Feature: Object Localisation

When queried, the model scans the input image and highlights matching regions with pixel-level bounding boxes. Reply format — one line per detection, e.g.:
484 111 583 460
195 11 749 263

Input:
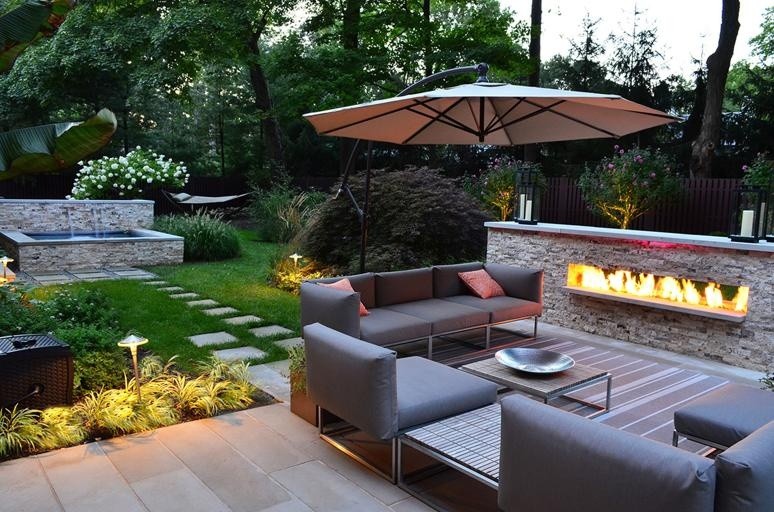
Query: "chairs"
302 320 498 485
498 381 774 512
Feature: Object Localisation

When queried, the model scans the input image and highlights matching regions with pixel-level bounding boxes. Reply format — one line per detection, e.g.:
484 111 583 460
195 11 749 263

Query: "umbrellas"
301 63 683 273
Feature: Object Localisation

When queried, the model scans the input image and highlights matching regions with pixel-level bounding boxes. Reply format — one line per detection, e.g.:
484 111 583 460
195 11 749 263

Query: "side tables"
397 402 501 512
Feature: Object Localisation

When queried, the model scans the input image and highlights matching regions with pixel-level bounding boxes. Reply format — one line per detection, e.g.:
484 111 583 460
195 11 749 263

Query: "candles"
519 193 532 221
758 202 766 237
740 209 754 236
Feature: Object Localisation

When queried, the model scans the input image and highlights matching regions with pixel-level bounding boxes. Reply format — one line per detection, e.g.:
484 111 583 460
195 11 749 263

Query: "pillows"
172 192 192 201
315 278 370 316
457 268 506 299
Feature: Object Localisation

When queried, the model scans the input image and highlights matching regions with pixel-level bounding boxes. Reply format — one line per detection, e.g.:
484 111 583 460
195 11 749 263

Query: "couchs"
301 261 545 361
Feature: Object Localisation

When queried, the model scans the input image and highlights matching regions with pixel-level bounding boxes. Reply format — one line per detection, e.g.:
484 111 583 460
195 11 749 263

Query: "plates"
495 347 575 375
